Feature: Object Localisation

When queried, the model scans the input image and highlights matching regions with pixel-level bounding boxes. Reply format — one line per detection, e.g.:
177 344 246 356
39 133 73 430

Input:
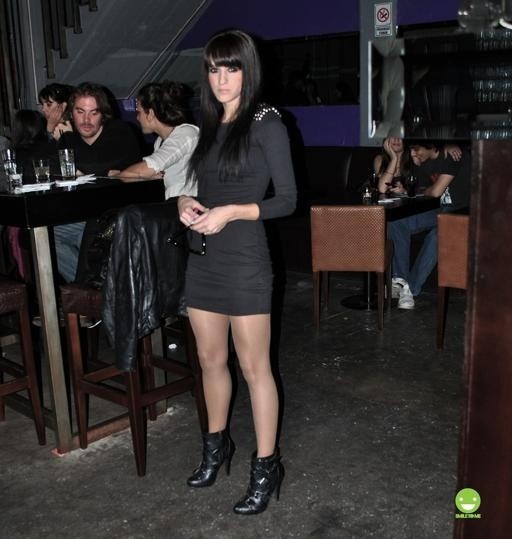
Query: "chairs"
310 205 394 330
437 213 469 351
59 200 208 477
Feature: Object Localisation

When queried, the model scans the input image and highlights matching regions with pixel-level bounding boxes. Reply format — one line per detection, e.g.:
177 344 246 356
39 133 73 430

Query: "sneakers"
385 277 407 299
79 315 102 328
32 306 65 327
397 284 415 310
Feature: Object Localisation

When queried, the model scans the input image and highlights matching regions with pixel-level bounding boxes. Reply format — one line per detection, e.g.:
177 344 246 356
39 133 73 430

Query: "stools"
0 282 46 445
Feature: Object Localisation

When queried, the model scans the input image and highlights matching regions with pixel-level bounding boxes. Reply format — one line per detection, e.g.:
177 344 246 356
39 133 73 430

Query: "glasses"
184 223 206 256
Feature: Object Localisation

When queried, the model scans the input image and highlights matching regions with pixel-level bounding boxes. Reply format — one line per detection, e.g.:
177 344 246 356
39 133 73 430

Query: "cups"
63 178 77 191
57 149 76 177
32 159 50 179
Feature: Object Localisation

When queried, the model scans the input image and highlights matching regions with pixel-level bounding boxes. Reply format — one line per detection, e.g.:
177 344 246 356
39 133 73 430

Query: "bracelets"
387 170 393 174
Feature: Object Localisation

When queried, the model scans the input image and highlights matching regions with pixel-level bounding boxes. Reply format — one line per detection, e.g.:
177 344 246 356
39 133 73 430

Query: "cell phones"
385 182 398 188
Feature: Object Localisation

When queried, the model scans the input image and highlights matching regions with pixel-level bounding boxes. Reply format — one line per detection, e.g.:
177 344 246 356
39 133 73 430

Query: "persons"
384 140 473 310
178 30 296 516
373 137 462 194
1 110 57 285
108 83 203 200
39 82 73 141
31 81 146 328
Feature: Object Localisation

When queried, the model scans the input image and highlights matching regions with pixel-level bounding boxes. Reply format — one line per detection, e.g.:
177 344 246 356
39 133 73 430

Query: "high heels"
233 445 285 515
186 429 235 488
